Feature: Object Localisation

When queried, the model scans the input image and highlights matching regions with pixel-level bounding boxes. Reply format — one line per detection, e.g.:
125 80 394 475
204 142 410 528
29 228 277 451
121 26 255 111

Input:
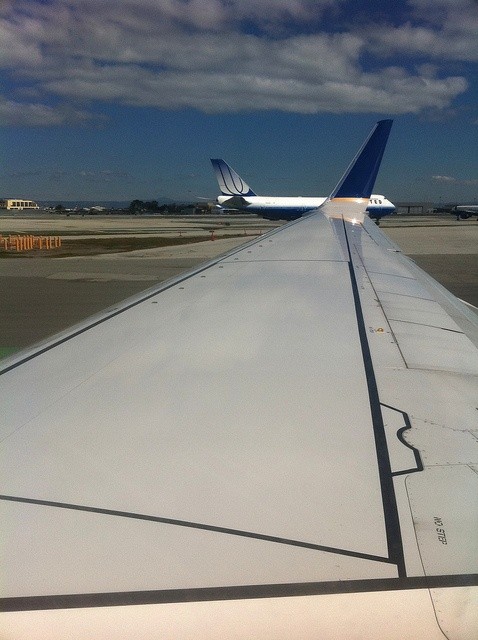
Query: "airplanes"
209 157 398 227
431 200 478 224
0 113 476 640
